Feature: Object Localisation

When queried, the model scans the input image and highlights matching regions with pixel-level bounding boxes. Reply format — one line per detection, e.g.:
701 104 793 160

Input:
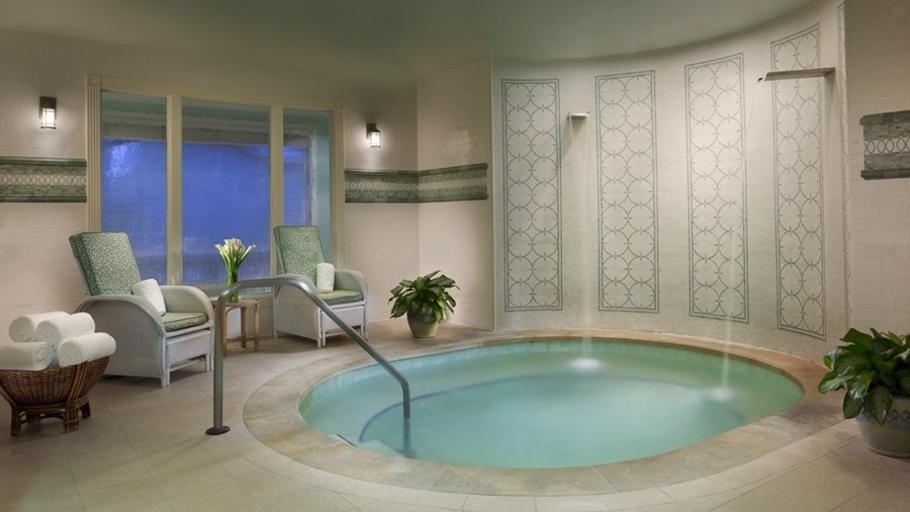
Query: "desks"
214 297 262 357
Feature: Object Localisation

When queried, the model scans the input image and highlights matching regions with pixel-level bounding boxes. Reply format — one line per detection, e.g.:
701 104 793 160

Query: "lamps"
363 120 386 153
36 94 61 132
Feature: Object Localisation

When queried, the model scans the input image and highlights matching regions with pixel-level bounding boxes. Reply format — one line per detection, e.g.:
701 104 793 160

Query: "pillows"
132 278 167 316
316 261 334 293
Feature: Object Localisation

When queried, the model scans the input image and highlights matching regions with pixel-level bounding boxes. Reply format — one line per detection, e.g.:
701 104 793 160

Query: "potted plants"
819 327 910 459
386 269 461 338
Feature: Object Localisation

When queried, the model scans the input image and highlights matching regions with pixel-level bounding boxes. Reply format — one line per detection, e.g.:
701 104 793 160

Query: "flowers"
213 237 256 266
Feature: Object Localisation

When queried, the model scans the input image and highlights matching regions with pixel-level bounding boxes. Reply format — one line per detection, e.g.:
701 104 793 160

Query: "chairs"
271 228 368 348
69 232 214 387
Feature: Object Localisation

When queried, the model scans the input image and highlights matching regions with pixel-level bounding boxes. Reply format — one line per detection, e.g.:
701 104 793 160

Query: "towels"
0 310 117 372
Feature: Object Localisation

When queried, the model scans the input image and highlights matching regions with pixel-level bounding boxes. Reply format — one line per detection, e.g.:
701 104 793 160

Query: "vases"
226 268 241 302
